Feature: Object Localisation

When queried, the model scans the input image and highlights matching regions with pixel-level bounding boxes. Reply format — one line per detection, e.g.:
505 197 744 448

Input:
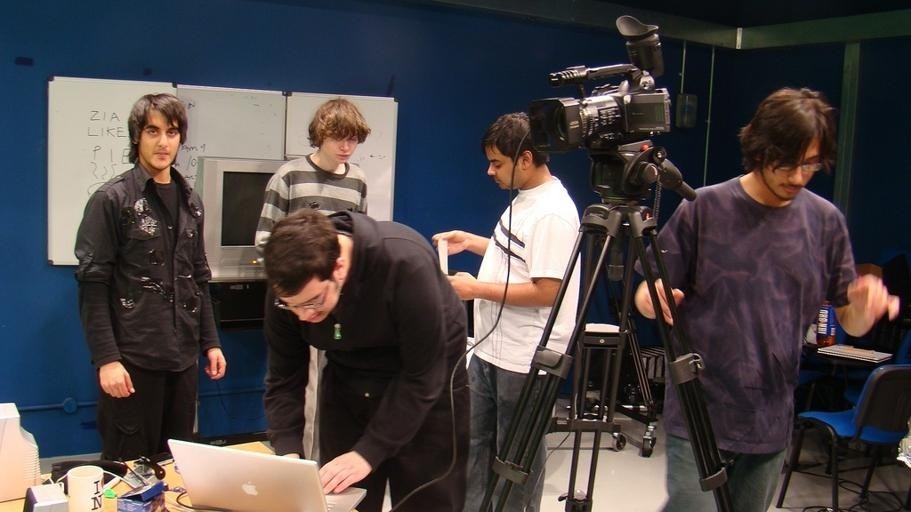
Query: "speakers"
675 94 698 129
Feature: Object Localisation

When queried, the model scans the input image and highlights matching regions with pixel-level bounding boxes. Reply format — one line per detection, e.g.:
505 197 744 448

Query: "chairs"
776 316 911 511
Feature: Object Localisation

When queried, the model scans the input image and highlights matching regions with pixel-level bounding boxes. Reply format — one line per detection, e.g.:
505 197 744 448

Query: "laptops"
167 436 367 512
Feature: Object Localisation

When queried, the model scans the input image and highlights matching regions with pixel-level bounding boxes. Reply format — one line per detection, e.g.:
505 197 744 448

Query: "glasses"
767 154 824 174
274 281 332 312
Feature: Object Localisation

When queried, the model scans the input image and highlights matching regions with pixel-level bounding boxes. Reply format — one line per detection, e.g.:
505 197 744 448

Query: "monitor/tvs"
195 157 291 280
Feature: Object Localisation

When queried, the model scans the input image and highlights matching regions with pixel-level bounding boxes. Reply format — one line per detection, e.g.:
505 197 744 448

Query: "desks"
1 439 278 512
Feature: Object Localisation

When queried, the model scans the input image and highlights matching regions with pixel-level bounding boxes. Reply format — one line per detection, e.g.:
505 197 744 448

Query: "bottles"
818 299 837 348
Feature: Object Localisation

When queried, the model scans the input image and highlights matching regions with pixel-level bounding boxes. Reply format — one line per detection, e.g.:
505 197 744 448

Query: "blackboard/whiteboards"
174 84 287 199
47 75 177 265
285 92 400 222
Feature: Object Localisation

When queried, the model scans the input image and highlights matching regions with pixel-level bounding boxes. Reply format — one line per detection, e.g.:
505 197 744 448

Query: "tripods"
478 203 736 512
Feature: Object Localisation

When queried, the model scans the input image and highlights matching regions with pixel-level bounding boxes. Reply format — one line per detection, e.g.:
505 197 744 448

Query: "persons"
255 98 370 458
264 208 474 511
635 87 901 511
76 93 230 459
434 109 586 510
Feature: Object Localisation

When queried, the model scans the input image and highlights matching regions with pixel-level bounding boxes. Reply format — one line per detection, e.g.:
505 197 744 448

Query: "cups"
67 466 105 511
